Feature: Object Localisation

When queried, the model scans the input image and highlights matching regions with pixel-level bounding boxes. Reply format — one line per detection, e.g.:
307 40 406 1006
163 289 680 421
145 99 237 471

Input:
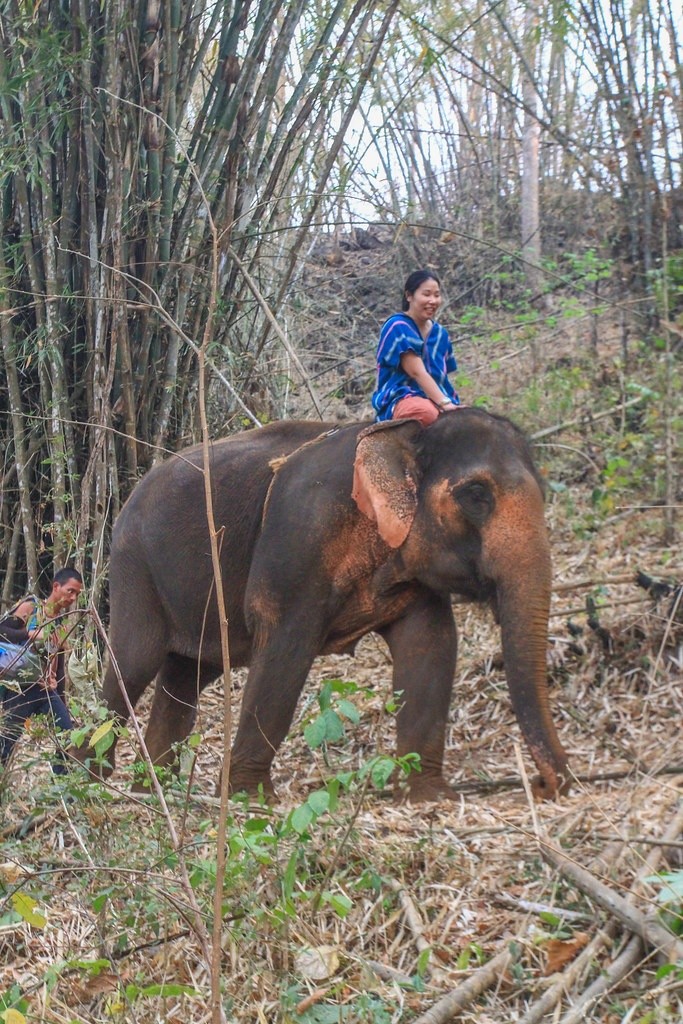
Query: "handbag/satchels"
0 594 45 686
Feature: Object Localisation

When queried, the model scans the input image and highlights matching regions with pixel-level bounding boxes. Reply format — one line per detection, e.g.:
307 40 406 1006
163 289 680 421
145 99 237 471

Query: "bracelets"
438 397 451 406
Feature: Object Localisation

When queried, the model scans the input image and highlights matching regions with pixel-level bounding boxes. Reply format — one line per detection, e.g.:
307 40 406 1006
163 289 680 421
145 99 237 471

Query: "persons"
0 567 82 773
370 270 471 421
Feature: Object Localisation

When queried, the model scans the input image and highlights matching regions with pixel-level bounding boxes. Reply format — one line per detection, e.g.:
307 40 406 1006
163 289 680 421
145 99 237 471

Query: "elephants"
66 408 574 815
